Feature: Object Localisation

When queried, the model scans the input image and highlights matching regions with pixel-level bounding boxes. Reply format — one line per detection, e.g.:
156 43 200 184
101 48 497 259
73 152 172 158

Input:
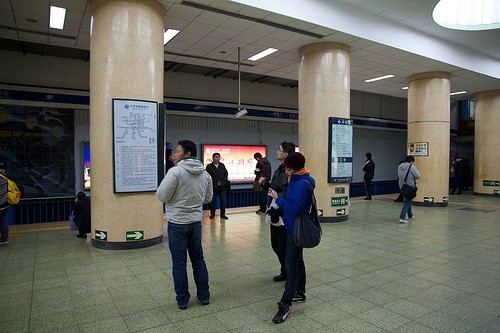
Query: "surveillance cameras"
235 109 248 118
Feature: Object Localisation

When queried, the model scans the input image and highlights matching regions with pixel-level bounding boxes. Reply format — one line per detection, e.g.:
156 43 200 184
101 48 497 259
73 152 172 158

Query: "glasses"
276 148 283 152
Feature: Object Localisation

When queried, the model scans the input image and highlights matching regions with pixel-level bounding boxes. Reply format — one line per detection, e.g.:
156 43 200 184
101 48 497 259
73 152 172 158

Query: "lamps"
235 47 248 118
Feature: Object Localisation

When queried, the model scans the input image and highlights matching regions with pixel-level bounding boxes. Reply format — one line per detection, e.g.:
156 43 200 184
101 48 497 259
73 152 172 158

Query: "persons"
206 153 228 219
166 149 175 174
73 192 91 238
398 155 421 223
268 152 315 323
157 140 214 310
363 152 375 200
268 142 295 282
254 153 271 214
0 176 10 246
449 156 471 195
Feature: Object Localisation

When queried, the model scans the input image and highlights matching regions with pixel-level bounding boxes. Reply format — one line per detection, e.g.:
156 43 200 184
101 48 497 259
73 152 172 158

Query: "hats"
282 152 305 170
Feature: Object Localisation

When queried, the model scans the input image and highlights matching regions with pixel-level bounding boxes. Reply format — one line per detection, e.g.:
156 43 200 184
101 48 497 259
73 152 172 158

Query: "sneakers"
273 305 290 323
290 292 306 302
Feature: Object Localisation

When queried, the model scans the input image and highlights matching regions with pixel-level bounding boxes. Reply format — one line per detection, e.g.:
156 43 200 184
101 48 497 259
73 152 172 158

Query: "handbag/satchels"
253 180 262 192
401 184 418 198
294 178 321 249
225 181 231 195
70 210 78 230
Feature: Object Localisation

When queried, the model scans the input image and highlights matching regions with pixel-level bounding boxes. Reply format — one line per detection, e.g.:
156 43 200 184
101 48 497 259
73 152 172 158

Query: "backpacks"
0 174 21 204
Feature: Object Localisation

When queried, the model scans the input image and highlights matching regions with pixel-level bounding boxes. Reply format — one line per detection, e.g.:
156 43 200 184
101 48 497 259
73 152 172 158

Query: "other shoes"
364 196 372 200
273 275 287 282
0 240 8 245
179 305 187 309
210 213 215 219
409 216 414 221
77 233 86 238
221 214 227 219
256 209 262 214
399 219 407 223
201 300 209 305
